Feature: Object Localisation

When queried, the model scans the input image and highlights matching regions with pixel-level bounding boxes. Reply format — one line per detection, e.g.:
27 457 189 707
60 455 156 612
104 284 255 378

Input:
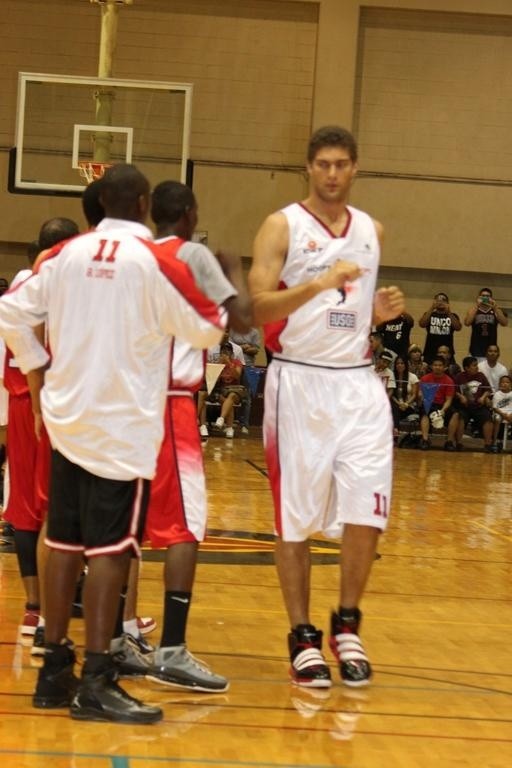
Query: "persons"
193 322 273 438
248 126 405 687
0 163 252 724
368 288 511 454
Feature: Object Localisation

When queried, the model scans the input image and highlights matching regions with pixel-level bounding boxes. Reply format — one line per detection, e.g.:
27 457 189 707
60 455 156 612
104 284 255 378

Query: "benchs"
200 398 243 438
400 415 512 453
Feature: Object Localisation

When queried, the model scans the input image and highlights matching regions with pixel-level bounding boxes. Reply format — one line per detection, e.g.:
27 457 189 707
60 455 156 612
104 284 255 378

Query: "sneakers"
288 624 331 688
70 666 161 723
327 608 373 686
33 666 78 709
421 439 430 449
109 633 156 679
200 417 249 438
21 572 157 656
446 441 500 453
147 643 230 692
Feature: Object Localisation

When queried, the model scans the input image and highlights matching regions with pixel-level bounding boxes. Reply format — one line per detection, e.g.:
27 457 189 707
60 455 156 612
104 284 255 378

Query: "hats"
408 344 422 355
380 351 393 362
221 342 233 350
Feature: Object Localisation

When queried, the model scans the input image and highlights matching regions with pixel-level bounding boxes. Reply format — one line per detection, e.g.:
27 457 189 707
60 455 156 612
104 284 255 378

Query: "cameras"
221 350 230 359
482 296 490 304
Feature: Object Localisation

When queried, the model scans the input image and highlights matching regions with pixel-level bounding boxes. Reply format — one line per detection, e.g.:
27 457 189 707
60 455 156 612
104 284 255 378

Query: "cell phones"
438 295 443 302
